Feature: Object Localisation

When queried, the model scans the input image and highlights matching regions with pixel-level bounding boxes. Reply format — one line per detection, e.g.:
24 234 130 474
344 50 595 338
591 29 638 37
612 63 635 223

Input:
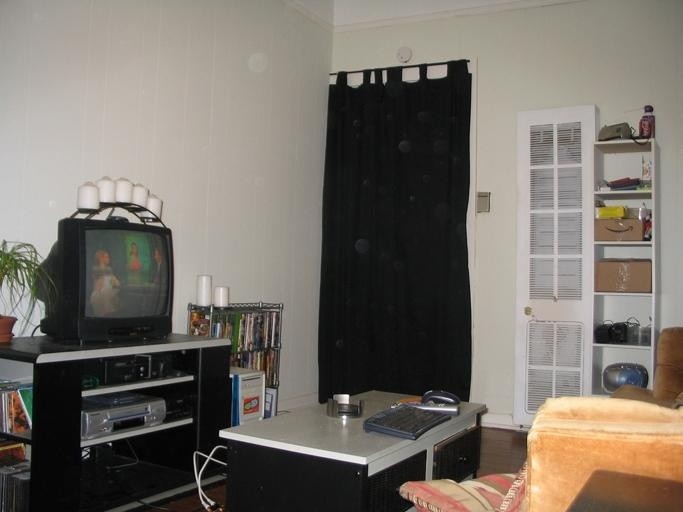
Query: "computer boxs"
229 366 265 427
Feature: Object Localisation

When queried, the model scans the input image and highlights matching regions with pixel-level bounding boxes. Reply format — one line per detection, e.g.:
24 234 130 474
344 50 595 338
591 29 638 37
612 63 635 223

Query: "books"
0 379 34 511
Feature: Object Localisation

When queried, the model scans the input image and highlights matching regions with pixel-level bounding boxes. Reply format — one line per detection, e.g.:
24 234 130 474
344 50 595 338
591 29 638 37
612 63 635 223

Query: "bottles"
638 104 655 138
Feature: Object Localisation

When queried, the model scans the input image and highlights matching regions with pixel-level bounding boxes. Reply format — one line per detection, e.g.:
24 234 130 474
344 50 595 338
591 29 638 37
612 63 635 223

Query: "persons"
125 240 145 280
90 249 122 315
149 244 168 284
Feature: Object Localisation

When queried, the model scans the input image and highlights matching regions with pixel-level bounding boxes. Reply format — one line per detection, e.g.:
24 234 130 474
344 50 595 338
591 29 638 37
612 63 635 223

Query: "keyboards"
364 406 451 440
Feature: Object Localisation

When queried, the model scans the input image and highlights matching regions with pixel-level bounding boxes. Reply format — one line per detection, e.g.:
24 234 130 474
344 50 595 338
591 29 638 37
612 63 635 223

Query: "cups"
77 176 163 219
196 275 229 308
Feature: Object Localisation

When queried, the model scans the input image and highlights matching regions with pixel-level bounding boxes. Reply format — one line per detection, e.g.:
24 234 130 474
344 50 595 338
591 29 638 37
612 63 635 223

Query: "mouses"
422 390 459 404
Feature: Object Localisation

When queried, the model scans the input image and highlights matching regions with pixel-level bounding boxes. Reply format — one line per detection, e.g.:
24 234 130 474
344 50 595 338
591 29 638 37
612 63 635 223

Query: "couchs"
520 327 683 512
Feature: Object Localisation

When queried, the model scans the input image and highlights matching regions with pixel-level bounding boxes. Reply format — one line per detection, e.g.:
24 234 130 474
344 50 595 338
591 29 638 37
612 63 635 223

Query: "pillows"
498 459 526 512
399 472 518 512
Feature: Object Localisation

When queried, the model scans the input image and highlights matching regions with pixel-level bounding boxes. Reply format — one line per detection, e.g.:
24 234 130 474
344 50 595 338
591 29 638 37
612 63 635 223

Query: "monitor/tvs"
34 218 173 344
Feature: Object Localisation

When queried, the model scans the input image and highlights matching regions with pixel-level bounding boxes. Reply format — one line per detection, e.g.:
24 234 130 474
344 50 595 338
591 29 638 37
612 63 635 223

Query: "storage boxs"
595 258 652 293
594 218 645 241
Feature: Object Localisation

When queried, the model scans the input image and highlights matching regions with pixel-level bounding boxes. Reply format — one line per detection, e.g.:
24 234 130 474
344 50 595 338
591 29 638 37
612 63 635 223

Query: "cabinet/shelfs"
594 139 662 397
516 106 600 427
0 334 231 512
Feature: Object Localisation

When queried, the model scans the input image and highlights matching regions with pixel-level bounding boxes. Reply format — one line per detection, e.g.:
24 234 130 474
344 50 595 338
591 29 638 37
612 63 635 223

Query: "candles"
115 177 133 202
77 181 100 209
214 287 229 307
132 182 149 208
140 194 163 222
96 177 117 203
195 275 211 306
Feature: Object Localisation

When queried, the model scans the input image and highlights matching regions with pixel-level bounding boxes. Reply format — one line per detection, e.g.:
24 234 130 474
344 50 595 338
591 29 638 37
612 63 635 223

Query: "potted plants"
0 238 58 343
